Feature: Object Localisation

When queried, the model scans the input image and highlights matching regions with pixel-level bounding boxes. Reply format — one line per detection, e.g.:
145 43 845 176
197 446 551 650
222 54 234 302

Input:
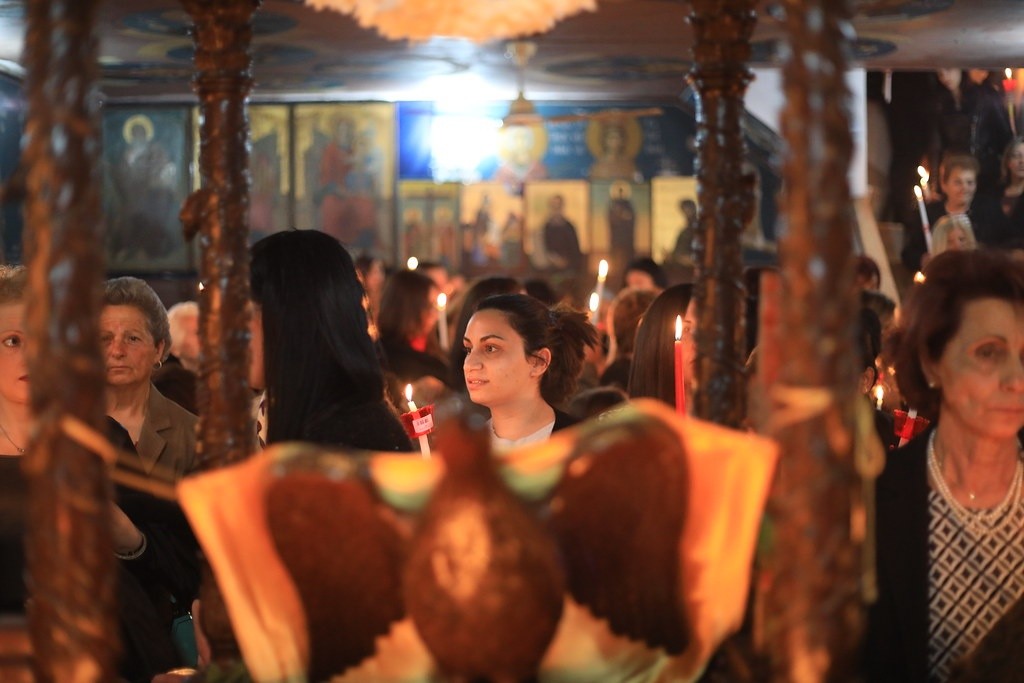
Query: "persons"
0 66 1024 683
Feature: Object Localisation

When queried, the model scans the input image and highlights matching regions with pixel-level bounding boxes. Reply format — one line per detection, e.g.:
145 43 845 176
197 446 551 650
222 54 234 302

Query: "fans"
401 42 664 126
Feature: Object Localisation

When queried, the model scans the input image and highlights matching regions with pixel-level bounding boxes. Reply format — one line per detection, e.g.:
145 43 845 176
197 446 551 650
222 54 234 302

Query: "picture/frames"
0 71 400 270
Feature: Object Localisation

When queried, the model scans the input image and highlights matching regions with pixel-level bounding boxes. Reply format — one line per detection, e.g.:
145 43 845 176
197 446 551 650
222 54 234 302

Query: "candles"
914 185 931 252
918 166 930 195
437 292 449 350
875 385 883 410
898 409 917 447
674 315 685 414
589 259 608 324
406 383 430 456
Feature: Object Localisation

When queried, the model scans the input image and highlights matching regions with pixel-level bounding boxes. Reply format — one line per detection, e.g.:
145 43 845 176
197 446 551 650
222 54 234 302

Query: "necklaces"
0 424 25 452
926 451 1023 533
929 428 1021 519
